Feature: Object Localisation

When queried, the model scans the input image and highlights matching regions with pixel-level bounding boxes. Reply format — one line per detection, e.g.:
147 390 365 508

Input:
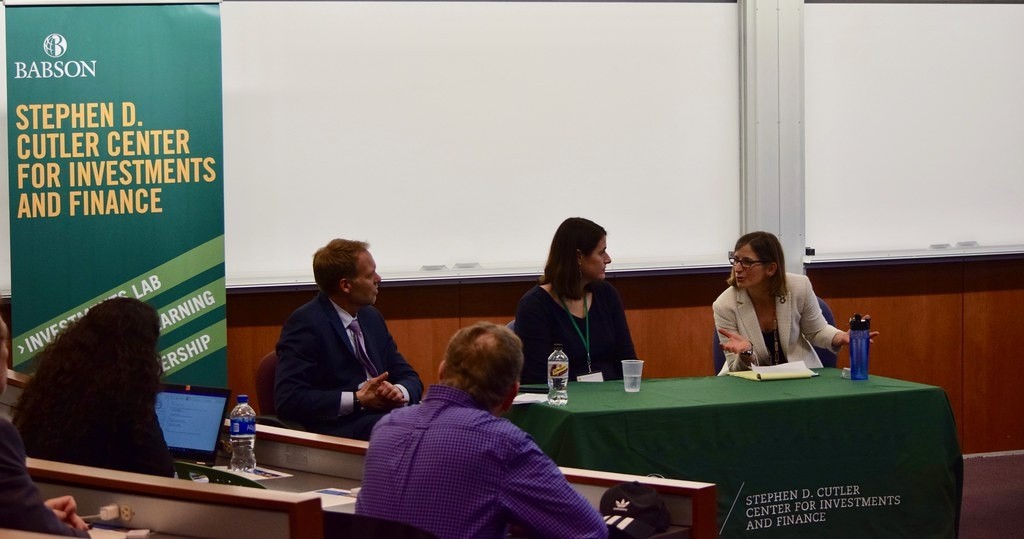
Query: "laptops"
154 383 232 466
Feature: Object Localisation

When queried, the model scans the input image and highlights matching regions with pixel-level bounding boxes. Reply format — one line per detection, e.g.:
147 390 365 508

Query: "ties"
348 320 377 377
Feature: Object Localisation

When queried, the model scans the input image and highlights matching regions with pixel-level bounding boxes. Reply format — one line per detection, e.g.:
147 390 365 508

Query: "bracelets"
354 394 364 413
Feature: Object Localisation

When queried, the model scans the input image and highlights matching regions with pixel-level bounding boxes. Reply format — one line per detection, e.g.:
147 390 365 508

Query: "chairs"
166 296 833 539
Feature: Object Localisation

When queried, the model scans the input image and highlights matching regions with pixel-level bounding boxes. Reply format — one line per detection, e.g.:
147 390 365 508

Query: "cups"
622 359 644 392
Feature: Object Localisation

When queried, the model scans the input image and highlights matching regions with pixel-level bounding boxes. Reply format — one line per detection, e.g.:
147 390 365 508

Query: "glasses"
729 257 761 267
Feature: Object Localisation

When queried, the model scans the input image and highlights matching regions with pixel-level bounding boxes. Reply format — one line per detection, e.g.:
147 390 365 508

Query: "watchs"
742 342 753 356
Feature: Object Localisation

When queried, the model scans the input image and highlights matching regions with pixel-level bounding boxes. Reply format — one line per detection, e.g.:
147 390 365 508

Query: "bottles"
230 395 256 472
547 344 569 406
850 313 870 380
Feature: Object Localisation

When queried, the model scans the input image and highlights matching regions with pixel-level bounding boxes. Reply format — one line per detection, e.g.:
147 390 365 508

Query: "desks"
0 370 719 539
504 366 963 539
23 457 323 538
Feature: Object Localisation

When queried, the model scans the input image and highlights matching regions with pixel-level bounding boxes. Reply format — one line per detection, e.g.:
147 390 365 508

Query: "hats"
599 481 670 539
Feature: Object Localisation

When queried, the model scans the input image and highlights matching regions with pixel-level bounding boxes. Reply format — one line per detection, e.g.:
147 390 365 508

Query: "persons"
270 239 425 440
514 218 637 381
712 231 880 376
0 317 93 539
13 298 176 475
356 322 608 539
